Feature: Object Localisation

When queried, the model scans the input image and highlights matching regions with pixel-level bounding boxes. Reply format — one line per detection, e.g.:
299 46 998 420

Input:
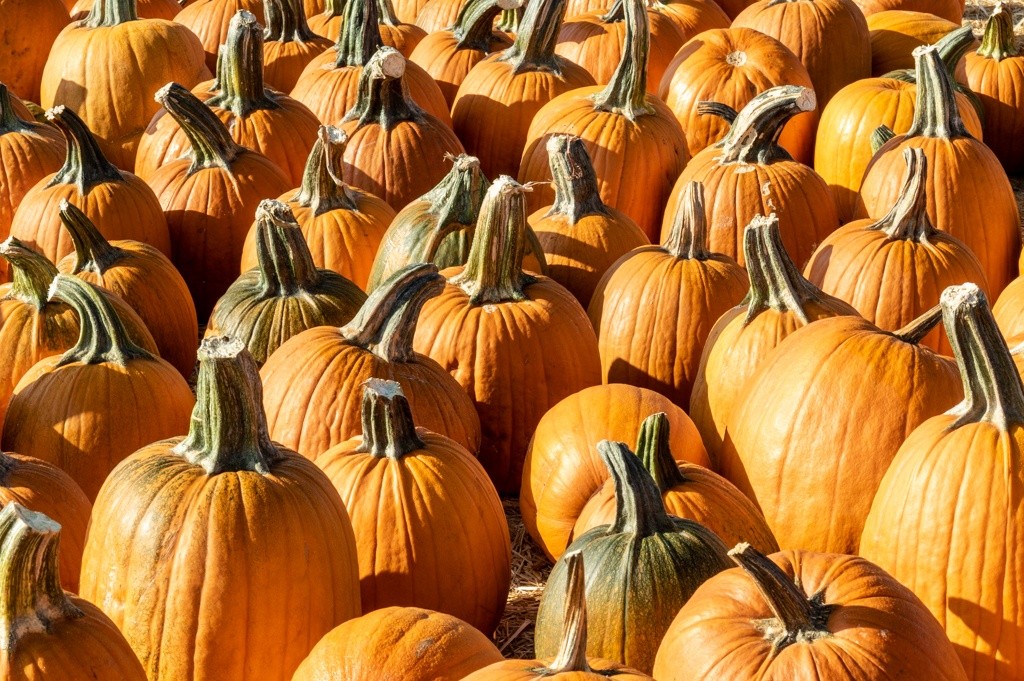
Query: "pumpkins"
0 1 1024 680
